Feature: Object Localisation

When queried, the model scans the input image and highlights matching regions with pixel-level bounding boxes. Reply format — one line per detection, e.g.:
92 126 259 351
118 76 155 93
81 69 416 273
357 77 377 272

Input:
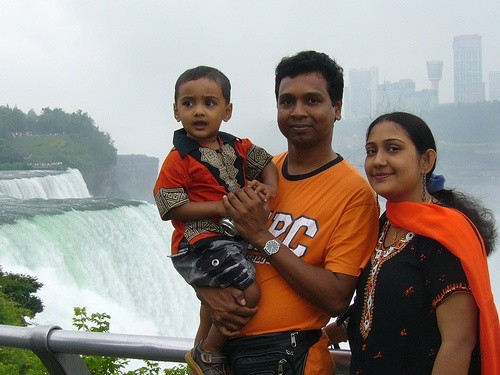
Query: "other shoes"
184 340 225 375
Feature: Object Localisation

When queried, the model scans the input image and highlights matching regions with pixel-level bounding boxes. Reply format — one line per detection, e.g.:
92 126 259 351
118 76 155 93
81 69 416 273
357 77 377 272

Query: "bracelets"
321 327 332 345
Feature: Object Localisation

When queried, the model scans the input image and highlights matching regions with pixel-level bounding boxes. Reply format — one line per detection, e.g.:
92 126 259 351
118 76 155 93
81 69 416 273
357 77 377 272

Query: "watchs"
260 236 282 258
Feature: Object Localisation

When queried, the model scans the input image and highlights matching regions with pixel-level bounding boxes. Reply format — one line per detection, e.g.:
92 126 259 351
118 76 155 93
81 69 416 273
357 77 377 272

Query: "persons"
192 50 381 375
155 66 279 375
321 112 500 374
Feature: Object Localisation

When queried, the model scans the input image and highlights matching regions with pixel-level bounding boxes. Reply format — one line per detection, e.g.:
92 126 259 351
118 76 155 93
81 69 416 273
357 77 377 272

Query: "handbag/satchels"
224 330 320 375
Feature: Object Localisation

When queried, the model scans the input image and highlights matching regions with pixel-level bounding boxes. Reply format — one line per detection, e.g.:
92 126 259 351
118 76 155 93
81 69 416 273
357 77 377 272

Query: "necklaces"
382 193 432 241
